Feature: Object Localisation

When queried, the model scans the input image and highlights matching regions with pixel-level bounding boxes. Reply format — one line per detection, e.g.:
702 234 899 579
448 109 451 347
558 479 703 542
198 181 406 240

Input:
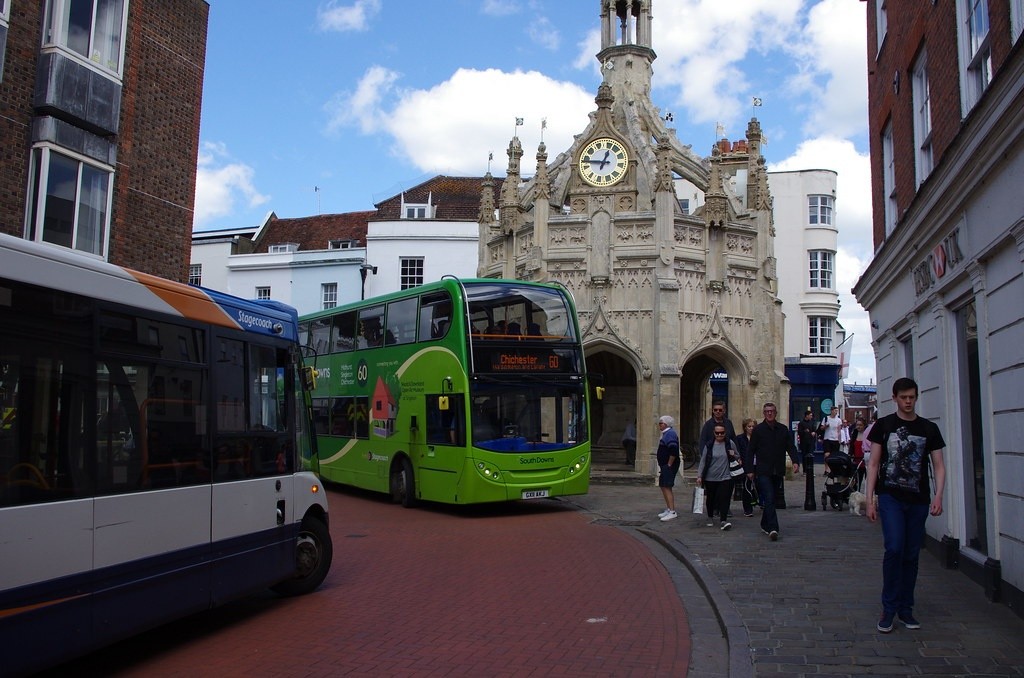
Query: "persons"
657 416 680 520
622 419 636 464
868 378 947 632
798 407 877 493
697 401 801 540
450 393 474 444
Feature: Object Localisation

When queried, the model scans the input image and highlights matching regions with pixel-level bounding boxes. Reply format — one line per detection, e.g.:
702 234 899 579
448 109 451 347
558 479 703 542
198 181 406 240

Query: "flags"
717 125 724 133
516 118 523 126
837 337 853 378
754 98 762 106
762 136 767 145
666 112 673 123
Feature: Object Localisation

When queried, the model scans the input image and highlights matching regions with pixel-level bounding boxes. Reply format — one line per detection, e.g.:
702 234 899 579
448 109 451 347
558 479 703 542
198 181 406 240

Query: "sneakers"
877 611 896 632
719 521 732 530
658 509 671 518
705 517 714 526
898 613 921 628
660 511 678 521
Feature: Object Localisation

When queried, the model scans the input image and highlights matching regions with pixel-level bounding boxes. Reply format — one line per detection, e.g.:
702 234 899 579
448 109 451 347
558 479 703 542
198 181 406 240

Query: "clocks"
579 137 629 188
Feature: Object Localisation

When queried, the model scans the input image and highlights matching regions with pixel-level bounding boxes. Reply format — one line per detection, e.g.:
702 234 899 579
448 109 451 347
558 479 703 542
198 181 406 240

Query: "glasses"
715 431 725 435
714 409 722 412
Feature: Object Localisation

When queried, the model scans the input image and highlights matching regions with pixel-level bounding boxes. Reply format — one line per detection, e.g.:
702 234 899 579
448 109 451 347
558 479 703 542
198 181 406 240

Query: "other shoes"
744 511 754 517
823 470 829 477
769 531 778 541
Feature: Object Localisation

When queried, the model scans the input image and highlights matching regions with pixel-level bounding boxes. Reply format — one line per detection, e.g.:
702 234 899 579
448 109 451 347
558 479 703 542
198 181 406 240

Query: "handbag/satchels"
692 481 704 514
728 454 744 476
816 416 828 439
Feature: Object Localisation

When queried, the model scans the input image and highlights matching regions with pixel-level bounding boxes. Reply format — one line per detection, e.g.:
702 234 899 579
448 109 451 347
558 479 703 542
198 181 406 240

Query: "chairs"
420 317 544 342
0 441 166 506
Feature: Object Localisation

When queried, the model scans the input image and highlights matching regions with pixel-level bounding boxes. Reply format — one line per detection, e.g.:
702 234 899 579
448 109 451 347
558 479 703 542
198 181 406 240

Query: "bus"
269 273 608 508
0 231 335 676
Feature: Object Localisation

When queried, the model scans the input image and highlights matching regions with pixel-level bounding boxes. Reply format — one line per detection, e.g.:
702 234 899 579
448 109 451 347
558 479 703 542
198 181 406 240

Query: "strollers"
817 451 866 510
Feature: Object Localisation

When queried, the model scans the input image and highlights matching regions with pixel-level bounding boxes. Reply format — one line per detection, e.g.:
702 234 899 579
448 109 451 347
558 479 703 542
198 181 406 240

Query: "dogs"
849 491 879 516
